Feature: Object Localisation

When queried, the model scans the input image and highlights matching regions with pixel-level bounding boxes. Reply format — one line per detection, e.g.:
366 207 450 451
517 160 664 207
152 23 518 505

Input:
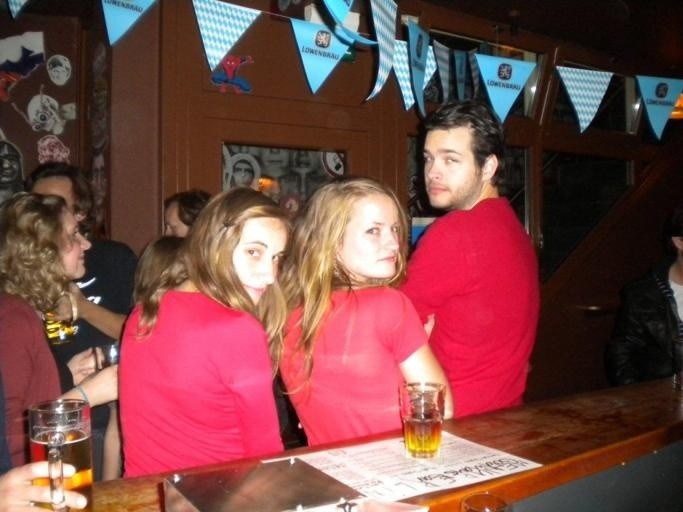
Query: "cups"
397 381 447 457
100 340 121 368
44 305 79 348
27 398 95 511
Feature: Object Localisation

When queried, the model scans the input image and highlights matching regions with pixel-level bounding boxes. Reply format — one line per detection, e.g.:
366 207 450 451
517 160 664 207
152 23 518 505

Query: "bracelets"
76 384 88 405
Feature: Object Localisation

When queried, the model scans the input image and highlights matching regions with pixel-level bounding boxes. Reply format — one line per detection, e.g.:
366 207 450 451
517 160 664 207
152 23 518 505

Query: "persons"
23 162 138 483
101 236 190 486
233 160 253 190
0 191 118 470
290 150 322 175
392 99 541 418
160 191 211 241
603 204 683 389
277 178 454 446
324 152 344 176
0 459 93 512
259 147 290 177
119 183 294 482
0 140 22 186
